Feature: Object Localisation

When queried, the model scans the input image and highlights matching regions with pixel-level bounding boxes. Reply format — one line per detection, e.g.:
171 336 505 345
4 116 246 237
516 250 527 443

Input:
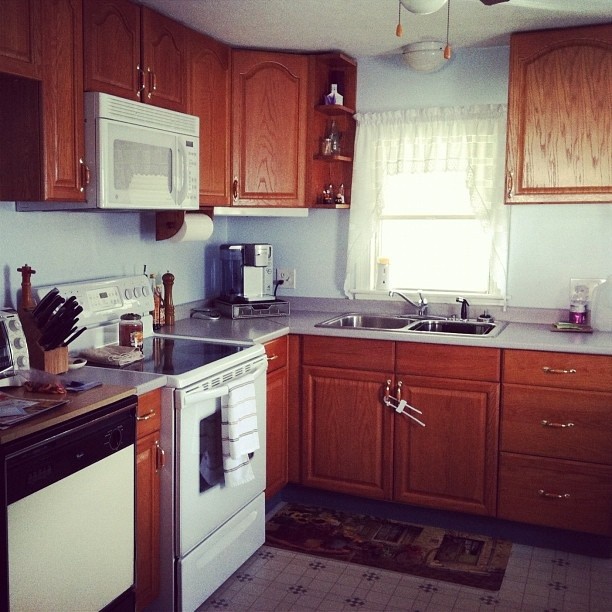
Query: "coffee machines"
220 241 275 302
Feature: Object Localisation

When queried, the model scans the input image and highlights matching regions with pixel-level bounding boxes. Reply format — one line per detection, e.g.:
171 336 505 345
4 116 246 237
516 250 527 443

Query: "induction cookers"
15 275 265 388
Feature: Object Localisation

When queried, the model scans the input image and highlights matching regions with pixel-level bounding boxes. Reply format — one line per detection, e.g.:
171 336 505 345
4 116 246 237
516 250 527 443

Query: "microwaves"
15 91 201 212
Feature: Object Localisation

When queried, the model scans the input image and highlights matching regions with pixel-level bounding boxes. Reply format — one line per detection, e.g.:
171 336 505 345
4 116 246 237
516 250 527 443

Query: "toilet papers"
166 214 214 242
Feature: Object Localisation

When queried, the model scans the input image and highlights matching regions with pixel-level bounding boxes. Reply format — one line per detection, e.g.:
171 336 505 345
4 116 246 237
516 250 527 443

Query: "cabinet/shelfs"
289 332 499 519
501 351 610 551
85 0 188 110
304 46 357 215
265 336 289 509
503 26 612 204
1 1 86 208
231 43 316 203
187 35 231 203
133 388 167 612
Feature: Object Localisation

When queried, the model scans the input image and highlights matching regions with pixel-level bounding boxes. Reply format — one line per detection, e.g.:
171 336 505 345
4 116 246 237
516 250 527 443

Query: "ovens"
159 355 266 611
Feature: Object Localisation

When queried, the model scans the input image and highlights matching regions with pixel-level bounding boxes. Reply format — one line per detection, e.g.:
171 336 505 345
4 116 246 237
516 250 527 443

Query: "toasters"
0 310 31 389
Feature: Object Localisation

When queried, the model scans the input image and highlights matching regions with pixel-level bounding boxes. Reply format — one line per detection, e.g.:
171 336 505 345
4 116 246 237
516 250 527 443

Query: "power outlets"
276 268 296 289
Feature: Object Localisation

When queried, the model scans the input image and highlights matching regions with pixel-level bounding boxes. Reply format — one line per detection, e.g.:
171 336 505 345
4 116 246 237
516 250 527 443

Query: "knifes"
32 287 87 350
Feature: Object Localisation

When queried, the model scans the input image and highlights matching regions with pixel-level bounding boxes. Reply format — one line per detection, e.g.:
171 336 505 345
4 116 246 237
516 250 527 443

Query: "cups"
376 258 390 291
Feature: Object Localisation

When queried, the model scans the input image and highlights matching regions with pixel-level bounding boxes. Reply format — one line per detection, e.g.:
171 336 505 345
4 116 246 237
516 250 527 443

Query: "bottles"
118 313 144 350
148 273 160 332
157 285 166 326
329 118 341 155
325 83 344 107
569 303 586 324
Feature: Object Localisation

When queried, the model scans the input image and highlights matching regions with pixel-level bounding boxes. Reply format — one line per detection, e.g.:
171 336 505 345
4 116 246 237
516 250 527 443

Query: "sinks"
314 313 417 337
408 318 511 337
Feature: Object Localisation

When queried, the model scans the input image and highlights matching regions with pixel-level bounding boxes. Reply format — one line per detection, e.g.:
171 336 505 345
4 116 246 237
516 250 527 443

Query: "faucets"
389 290 431 320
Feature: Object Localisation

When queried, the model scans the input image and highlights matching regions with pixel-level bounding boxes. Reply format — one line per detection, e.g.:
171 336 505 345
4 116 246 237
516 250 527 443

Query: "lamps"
398 0 448 17
403 40 453 74
